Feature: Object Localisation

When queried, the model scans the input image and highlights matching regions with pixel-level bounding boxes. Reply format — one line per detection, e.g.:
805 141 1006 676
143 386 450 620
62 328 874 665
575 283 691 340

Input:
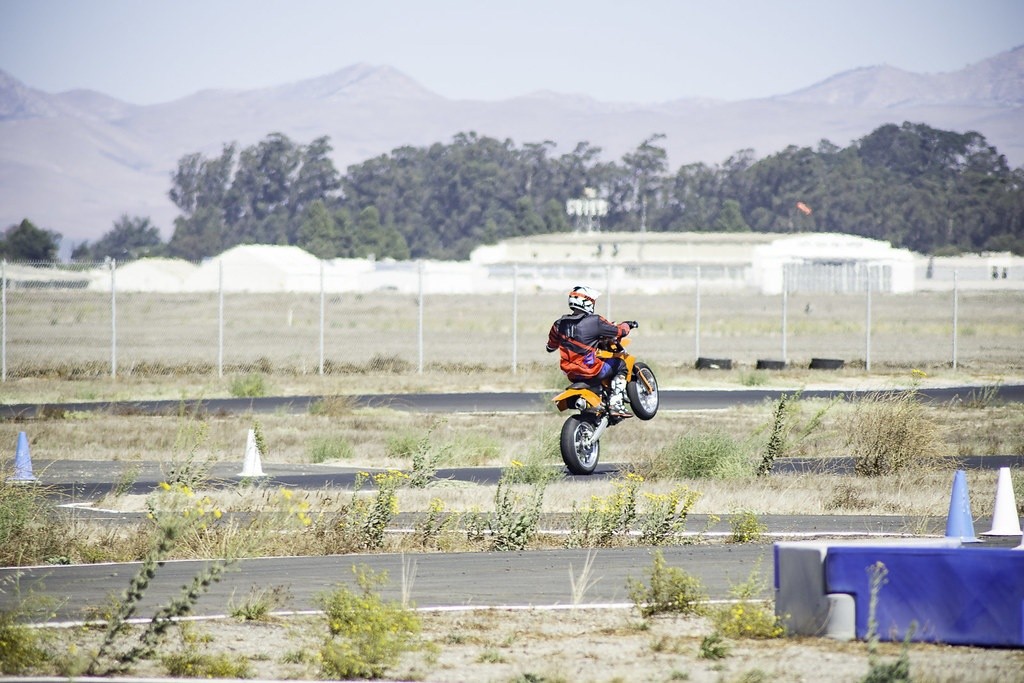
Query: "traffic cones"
2 430 38 481
945 470 987 544
979 466 1024 537
1011 529 1024 551
236 429 268 477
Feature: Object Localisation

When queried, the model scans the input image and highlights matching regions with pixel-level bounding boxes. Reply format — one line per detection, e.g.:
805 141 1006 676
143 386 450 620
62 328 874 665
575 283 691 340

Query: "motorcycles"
549 320 661 475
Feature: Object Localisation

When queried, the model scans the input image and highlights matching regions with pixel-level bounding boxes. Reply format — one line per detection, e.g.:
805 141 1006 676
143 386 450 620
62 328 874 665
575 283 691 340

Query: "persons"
545 285 634 415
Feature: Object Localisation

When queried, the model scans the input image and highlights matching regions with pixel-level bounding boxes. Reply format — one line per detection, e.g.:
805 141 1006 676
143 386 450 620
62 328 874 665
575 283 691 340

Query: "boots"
609 375 634 417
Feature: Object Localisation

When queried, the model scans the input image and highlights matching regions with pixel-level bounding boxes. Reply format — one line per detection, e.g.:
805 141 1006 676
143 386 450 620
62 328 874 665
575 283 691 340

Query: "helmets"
569 285 603 314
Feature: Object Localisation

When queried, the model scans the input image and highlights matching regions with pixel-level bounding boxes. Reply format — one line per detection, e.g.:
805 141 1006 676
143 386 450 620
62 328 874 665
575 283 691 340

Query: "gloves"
625 321 635 328
